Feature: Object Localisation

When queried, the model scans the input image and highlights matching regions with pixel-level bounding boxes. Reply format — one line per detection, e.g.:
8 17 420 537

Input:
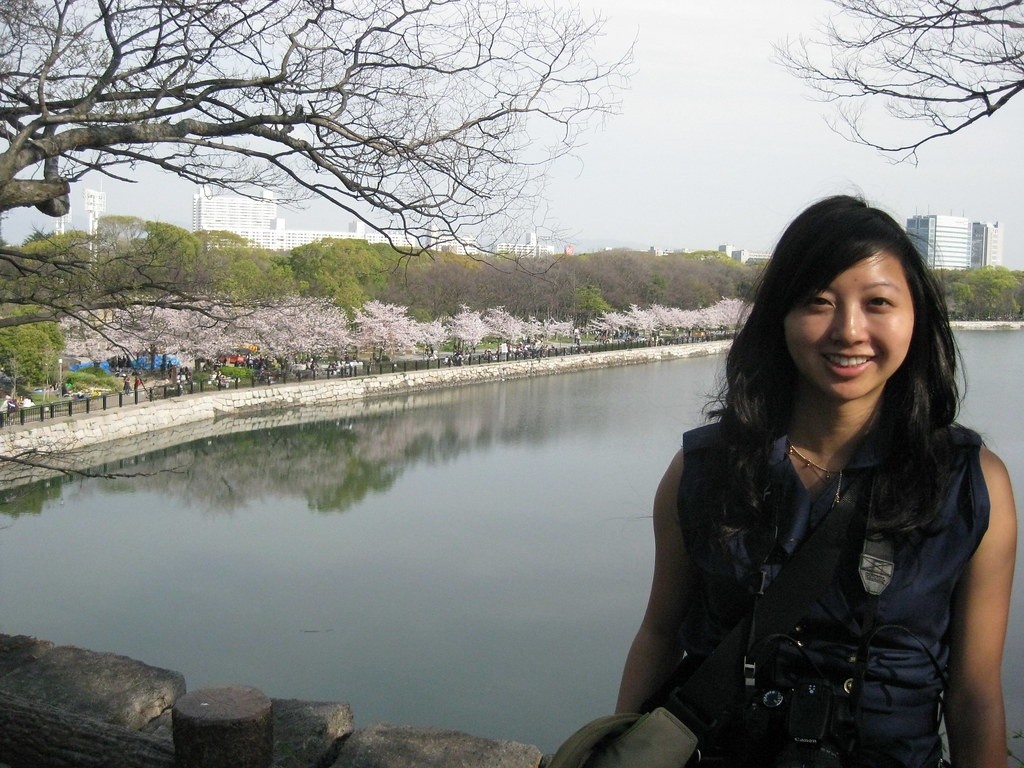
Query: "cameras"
727 640 861 768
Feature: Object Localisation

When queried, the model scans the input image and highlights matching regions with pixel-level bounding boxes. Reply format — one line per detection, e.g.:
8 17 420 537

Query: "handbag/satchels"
537 710 700 768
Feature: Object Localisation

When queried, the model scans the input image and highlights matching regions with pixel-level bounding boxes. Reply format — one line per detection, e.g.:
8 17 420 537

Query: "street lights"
57 358 63 399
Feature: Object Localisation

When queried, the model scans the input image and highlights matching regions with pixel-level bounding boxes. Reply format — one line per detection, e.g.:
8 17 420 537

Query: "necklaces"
786 438 839 480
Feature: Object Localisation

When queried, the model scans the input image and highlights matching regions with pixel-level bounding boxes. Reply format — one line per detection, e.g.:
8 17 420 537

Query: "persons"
5 393 26 428
168 327 726 396
614 192 1017 766
134 376 141 391
123 376 132 394
